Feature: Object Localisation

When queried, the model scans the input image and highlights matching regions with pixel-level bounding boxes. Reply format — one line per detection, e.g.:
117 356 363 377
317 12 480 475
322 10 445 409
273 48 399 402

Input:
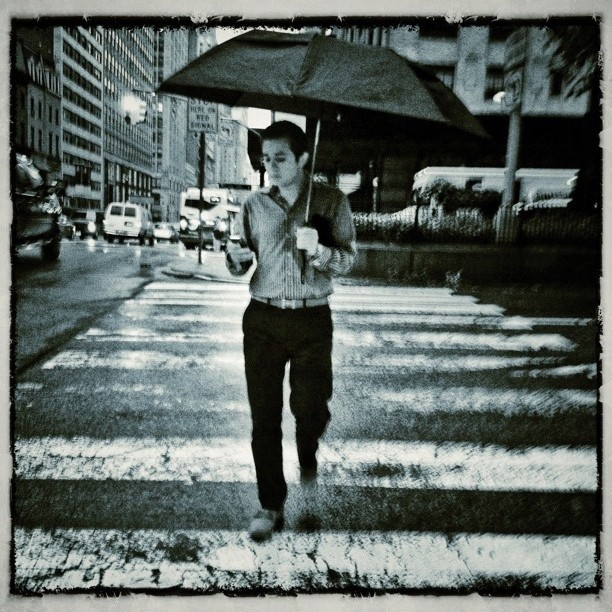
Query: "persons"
222 121 360 542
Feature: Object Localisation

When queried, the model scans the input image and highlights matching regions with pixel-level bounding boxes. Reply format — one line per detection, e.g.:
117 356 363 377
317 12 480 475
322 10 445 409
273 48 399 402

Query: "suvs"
10 153 68 268
66 210 104 241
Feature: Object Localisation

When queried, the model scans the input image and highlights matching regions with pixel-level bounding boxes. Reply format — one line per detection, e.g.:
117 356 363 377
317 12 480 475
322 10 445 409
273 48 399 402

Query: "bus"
178 187 252 251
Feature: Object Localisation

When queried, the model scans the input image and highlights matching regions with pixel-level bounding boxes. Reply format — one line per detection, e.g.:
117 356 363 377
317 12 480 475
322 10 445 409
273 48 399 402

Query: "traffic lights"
119 93 146 126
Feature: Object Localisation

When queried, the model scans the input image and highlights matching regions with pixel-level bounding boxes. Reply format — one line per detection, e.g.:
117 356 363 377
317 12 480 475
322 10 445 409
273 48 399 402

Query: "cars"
152 224 178 245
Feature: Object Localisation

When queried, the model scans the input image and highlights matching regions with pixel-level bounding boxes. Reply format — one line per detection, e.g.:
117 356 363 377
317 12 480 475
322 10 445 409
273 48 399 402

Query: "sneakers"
248 508 285 538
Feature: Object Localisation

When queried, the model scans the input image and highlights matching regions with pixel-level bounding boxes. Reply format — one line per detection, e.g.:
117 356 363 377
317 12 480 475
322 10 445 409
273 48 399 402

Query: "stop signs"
186 96 219 134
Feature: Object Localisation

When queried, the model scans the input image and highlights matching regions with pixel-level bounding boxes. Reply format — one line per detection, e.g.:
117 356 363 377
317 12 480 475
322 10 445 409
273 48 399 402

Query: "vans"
102 200 155 247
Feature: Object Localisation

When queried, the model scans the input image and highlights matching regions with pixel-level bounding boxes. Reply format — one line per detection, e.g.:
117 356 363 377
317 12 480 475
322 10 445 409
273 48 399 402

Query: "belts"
250 294 328 310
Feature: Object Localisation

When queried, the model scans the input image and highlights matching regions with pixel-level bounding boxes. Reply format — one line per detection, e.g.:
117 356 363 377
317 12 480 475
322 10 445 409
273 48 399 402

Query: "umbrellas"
153 30 493 284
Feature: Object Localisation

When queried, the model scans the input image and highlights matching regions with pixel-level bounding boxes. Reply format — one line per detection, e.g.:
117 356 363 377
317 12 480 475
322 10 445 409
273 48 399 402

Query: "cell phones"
228 236 253 268
